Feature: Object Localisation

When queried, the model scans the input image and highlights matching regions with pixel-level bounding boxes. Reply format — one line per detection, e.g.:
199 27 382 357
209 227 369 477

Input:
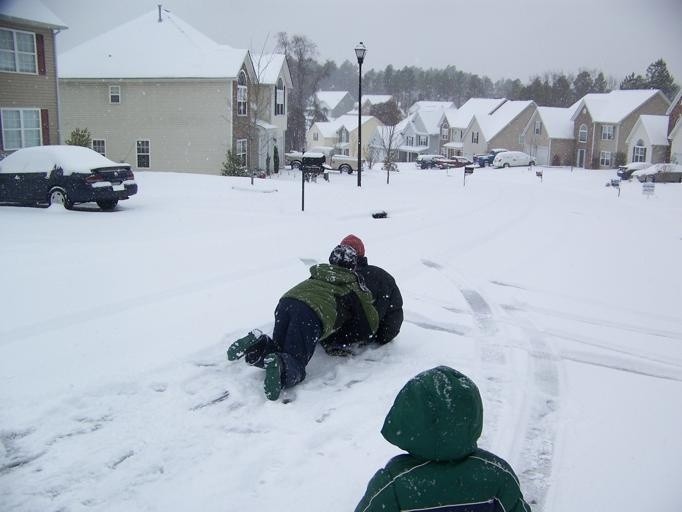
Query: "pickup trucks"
436 156 470 169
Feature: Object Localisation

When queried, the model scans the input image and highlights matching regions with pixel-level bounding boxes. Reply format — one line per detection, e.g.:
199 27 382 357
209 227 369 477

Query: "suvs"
479 149 507 167
300 153 326 175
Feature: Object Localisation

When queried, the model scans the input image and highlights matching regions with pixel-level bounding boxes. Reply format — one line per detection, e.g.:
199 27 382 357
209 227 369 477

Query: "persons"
353 365 531 511
324 234 403 354
224 243 382 404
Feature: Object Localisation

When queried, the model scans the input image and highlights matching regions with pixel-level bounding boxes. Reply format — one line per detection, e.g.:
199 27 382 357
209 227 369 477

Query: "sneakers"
264 353 284 401
227 329 262 360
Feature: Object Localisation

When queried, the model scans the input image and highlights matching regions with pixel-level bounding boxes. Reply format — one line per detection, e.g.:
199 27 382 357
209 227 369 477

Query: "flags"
611 178 622 189
465 165 475 175
535 168 544 177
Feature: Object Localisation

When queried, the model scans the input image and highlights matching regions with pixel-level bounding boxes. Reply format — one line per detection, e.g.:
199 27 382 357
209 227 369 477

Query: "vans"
416 154 446 169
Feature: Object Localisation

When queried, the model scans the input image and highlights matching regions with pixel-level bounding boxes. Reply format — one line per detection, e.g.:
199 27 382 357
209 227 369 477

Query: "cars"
617 161 682 183
493 151 535 167
1 145 137 211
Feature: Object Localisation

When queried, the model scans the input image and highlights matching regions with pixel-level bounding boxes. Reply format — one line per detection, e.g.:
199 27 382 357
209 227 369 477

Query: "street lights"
354 42 366 188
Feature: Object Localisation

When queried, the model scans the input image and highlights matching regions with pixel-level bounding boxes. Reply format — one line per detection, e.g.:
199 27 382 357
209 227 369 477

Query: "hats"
329 245 356 270
341 234 364 257
